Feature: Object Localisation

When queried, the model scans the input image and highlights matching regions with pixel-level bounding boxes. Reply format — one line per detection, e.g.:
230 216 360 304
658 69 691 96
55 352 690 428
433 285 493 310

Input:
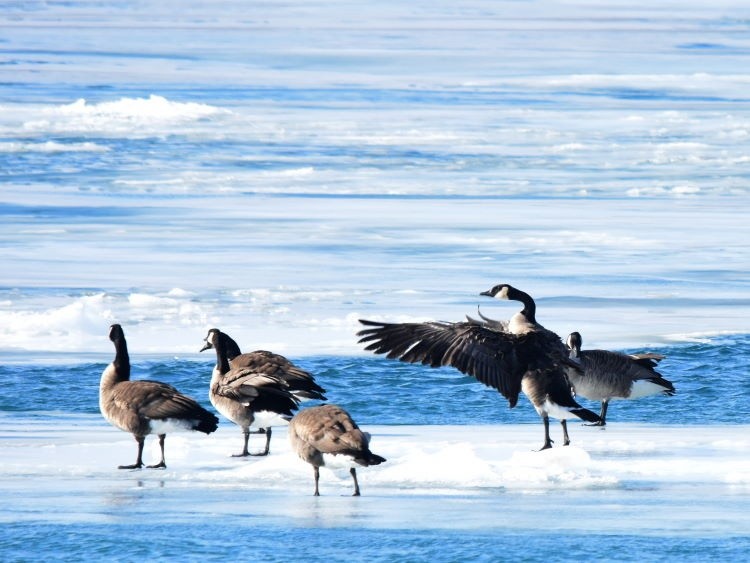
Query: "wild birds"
290 404 387 495
98 324 220 469
356 284 603 451
200 332 329 433
566 332 676 427
204 329 301 458
